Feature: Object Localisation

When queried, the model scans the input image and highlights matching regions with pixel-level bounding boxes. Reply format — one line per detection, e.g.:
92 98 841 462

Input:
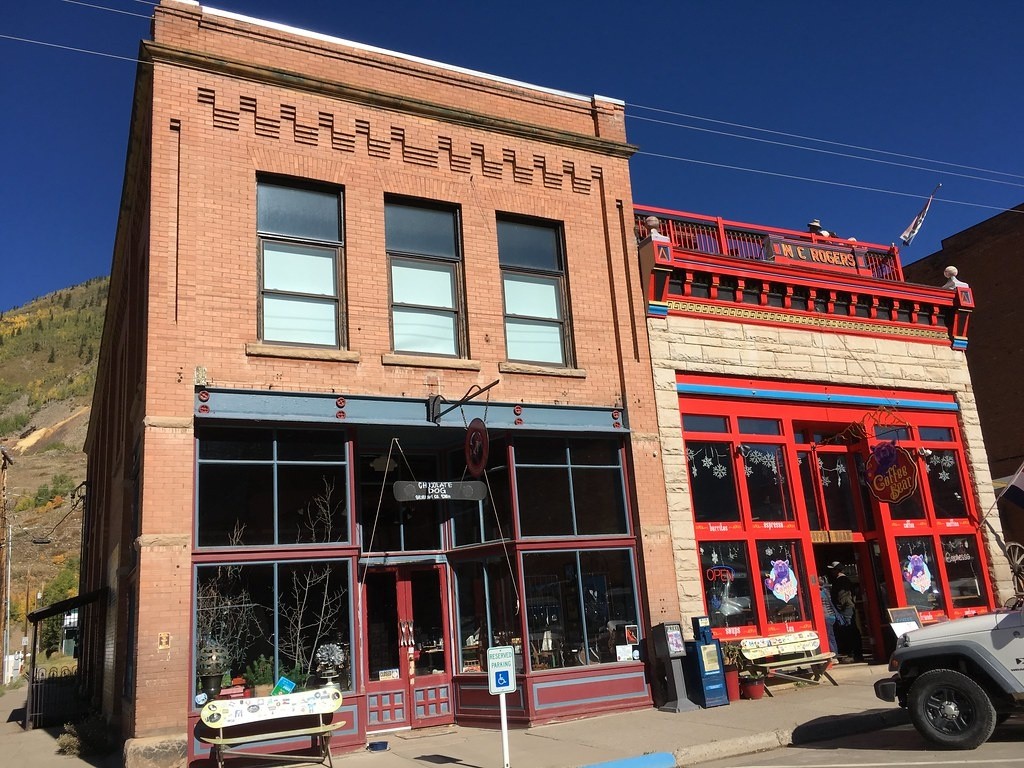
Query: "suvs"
874 605 1024 750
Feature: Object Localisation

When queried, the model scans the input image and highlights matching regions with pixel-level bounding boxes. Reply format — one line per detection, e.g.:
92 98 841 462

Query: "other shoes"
842 657 865 663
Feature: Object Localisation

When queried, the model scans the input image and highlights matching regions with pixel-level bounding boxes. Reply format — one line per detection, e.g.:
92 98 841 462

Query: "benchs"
741 629 839 697
200 687 348 768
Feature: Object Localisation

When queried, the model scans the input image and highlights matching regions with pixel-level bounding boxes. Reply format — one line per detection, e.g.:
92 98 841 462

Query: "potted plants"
719 641 744 700
736 662 771 699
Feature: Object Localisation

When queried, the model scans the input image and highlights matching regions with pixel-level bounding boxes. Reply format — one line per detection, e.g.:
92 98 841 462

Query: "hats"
806 218 822 229
826 561 844 570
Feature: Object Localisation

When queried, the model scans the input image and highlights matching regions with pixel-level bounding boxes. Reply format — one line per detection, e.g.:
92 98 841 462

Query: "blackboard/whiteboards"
887 606 923 629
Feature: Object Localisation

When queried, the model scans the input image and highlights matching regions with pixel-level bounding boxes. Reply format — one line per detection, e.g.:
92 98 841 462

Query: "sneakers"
832 659 839 665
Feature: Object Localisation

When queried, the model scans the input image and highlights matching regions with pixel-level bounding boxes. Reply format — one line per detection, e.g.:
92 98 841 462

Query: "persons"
818 575 839 664
832 575 866 661
801 220 833 244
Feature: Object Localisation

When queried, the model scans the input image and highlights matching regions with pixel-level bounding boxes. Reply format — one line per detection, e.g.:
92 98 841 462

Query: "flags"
899 195 933 246
1013 468 1024 490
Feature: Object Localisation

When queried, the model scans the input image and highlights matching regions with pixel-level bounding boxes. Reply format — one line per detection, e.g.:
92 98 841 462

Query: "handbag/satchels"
836 614 847 627
